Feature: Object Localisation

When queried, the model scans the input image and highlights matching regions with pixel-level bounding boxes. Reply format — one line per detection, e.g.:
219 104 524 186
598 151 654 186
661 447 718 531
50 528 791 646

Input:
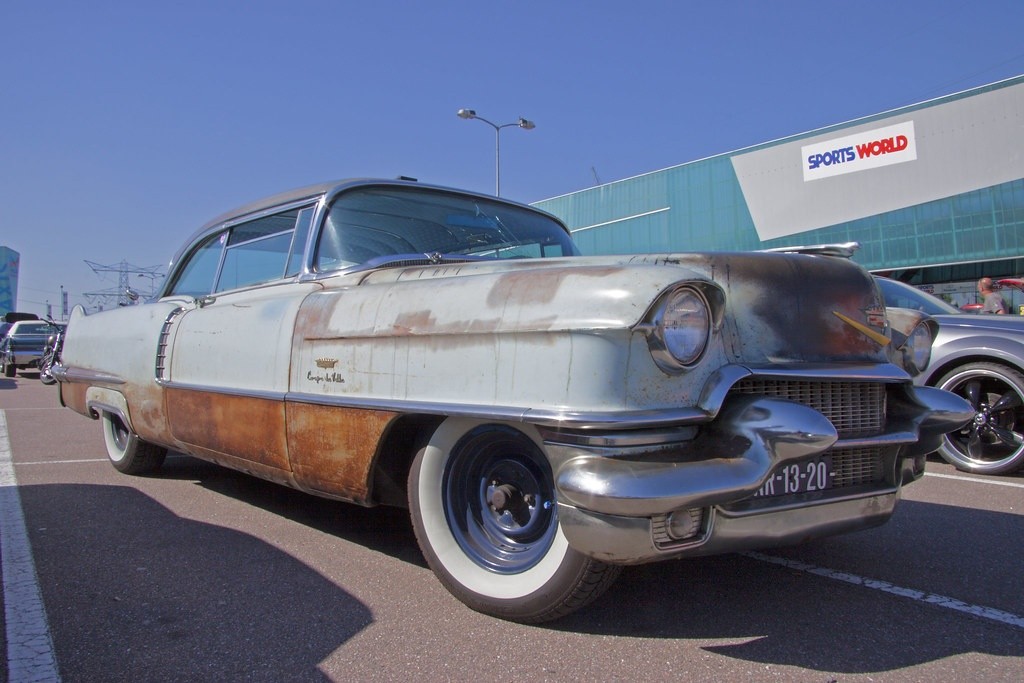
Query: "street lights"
458 106 536 197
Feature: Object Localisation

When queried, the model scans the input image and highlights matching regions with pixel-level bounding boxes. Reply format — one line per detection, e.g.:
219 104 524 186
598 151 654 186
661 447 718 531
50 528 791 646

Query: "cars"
869 275 1023 476
0 312 66 378
50 177 976 626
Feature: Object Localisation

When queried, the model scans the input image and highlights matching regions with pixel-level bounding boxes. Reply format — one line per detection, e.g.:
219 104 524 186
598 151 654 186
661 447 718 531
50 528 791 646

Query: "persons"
978 278 1010 317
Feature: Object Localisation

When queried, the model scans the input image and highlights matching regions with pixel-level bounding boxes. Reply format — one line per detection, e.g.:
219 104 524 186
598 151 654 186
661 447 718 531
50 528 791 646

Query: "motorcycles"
38 314 65 385
